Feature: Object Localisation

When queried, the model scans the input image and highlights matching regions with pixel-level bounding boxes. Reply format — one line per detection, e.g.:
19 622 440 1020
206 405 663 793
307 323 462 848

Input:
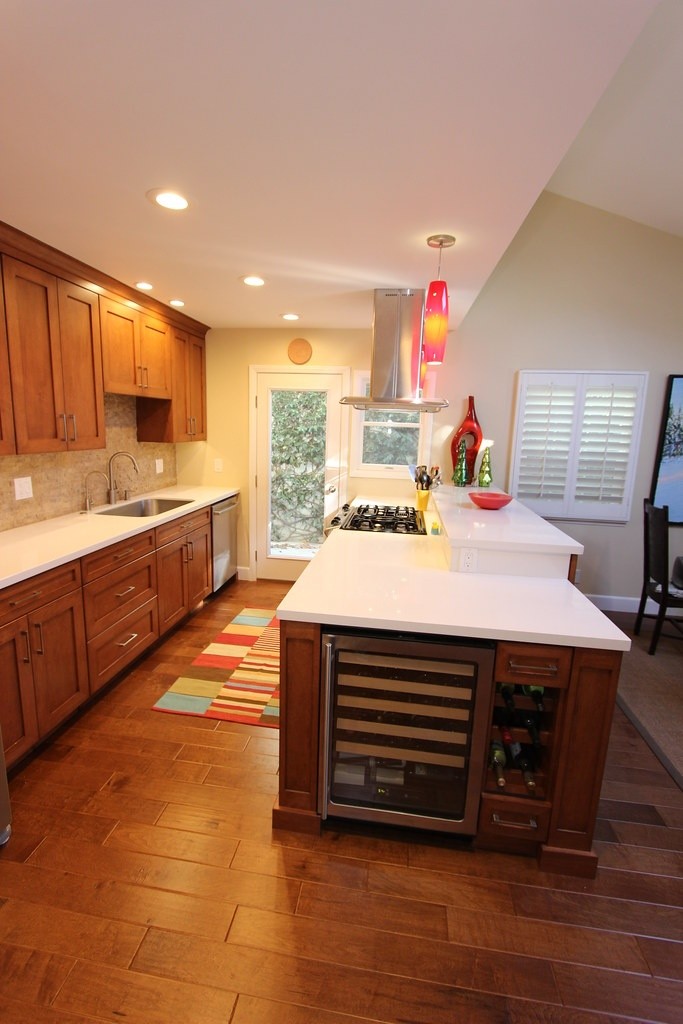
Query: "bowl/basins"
468 491 513 510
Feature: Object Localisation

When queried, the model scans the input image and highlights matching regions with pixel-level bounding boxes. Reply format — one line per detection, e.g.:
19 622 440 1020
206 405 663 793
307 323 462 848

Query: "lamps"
422 234 457 366
419 364 428 391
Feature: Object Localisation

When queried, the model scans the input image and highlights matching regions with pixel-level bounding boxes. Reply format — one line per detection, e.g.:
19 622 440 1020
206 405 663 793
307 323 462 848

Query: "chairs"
633 497 683 655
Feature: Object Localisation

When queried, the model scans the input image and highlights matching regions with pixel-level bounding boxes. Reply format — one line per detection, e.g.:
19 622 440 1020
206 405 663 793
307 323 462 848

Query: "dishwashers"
210 495 240 592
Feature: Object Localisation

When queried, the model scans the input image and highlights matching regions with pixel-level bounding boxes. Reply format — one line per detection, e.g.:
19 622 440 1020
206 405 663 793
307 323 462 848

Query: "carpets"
612 628 683 794
151 606 279 729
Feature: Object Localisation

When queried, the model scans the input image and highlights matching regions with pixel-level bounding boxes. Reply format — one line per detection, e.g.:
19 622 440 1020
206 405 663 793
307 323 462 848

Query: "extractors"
339 289 449 413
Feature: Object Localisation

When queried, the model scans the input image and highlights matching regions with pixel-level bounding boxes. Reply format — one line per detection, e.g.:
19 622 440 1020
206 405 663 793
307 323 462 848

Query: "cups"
416 490 430 511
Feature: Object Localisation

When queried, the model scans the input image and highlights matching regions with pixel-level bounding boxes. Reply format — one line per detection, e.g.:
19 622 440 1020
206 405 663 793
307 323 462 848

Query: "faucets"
107 452 140 504
81 470 111 511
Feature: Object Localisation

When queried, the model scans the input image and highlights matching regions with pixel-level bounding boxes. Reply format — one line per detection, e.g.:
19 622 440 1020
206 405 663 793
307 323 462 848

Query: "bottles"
450 396 483 485
478 446 493 488
453 439 471 487
335 667 461 801
487 684 546 792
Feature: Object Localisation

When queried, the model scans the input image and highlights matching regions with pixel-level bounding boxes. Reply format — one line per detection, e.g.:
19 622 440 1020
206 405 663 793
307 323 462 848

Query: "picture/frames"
648 374 683 528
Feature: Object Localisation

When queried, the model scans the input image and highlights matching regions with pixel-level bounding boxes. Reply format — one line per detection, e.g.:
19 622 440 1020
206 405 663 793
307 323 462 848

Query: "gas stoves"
330 503 427 535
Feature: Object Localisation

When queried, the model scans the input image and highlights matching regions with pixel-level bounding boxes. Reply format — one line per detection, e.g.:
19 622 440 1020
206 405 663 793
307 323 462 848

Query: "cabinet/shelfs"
1 252 210 454
0 556 91 773
154 505 213 637
470 639 575 854
79 527 160 695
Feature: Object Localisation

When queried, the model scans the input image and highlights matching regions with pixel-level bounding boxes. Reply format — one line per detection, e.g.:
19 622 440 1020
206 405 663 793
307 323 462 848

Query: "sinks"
92 497 196 518
